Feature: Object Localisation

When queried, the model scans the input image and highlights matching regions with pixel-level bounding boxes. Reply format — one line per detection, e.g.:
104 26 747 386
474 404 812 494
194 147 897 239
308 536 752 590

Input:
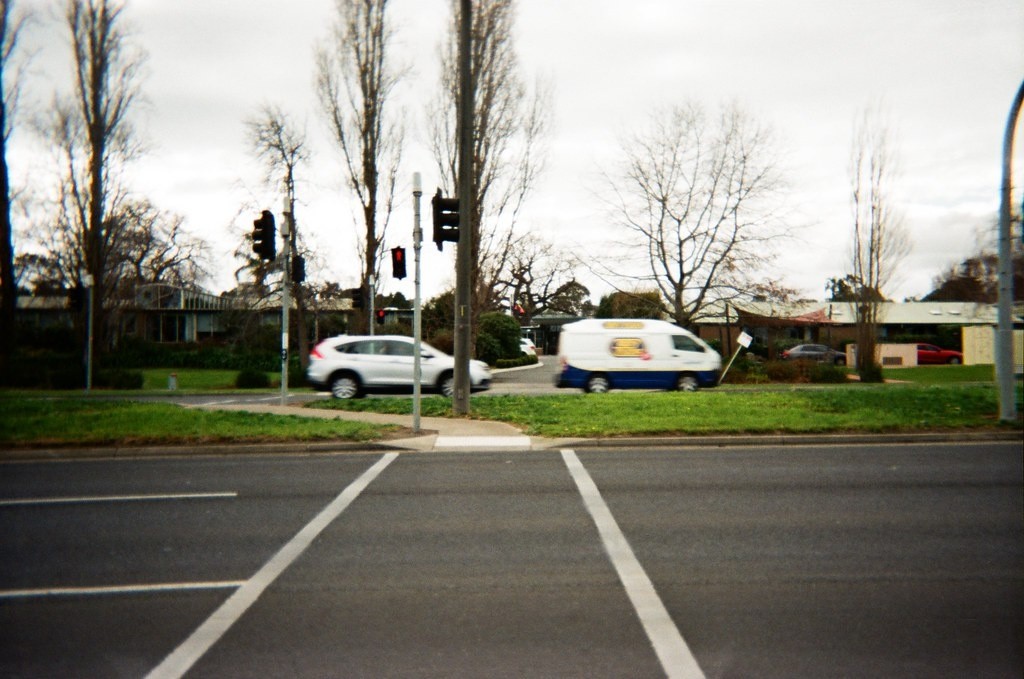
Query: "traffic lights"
352 286 364 310
252 210 276 261
432 193 460 250
377 309 385 325
392 246 406 277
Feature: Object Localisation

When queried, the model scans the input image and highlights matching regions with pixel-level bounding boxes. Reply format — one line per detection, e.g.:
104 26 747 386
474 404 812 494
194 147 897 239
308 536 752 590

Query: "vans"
554 318 723 391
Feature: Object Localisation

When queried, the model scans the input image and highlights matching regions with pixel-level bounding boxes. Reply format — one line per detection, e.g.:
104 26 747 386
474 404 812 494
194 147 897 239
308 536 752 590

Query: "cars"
306 334 493 400
519 337 537 357
915 342 963 366
783 344 847 368
